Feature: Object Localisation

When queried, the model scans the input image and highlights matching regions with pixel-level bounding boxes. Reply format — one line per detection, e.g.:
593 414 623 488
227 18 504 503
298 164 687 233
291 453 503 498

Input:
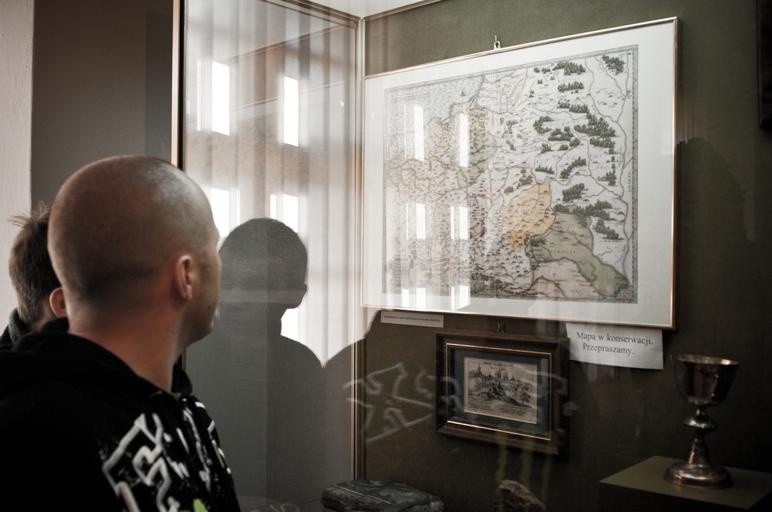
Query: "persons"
1 156 243 512
1 205 67 360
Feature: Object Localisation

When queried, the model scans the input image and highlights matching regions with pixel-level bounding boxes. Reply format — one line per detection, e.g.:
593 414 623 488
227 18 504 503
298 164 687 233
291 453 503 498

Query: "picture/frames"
430 328 570 461
357 13 678 336
